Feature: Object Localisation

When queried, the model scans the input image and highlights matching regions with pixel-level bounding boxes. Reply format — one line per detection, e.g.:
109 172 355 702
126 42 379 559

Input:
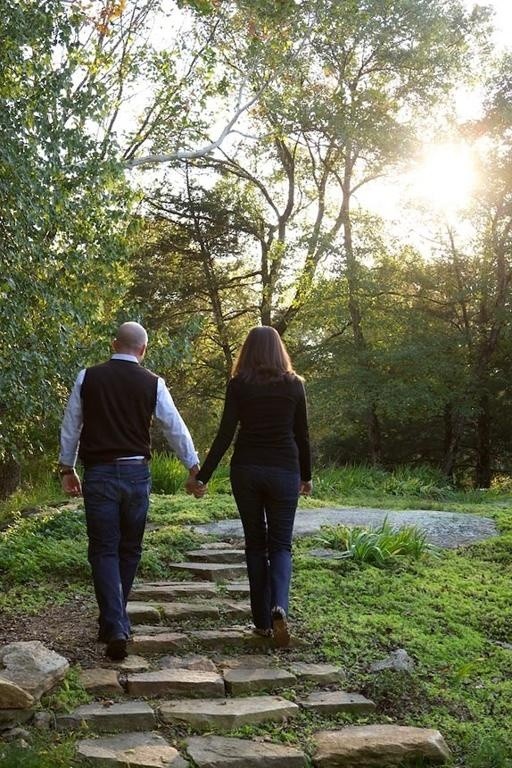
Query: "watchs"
60 468 75 476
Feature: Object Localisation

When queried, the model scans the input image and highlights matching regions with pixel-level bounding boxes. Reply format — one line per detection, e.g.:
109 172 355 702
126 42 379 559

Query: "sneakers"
254 606 291 647
97 626 127 662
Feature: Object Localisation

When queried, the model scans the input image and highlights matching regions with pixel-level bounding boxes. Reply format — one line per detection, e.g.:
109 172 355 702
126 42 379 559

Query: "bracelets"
198 480 204 485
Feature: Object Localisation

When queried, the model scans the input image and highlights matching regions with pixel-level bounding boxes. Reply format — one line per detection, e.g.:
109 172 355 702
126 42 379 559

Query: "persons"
59 321 207 662
196 325 313 646
